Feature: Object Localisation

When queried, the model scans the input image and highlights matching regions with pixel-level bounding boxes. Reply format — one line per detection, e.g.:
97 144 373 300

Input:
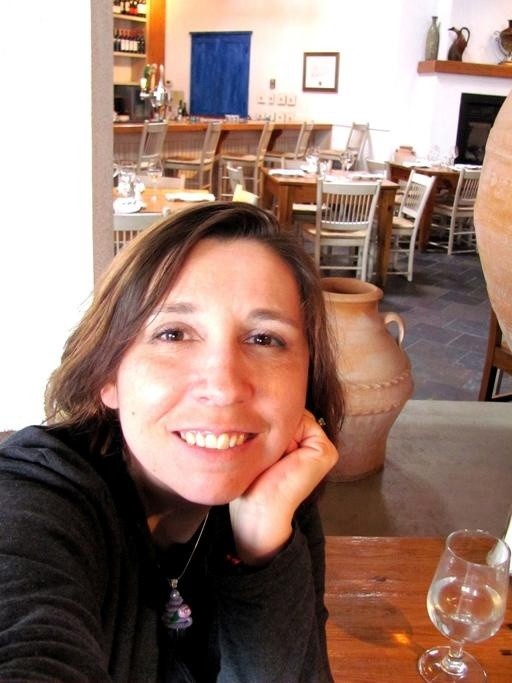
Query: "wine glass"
148 158 164 192
428 144 459 171
417 529 511 683
120 160 138 197
305 146 354 182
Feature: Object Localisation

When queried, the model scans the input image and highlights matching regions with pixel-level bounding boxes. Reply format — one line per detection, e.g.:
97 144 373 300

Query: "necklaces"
151 504 210 632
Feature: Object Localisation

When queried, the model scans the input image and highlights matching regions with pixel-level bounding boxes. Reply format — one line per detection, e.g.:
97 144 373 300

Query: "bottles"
113 28 145 54
112 0 147 19
140 63 166 100
177 100 189 122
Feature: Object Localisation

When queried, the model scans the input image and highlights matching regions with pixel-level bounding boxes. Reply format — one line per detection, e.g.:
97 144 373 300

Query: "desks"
322 535 511 683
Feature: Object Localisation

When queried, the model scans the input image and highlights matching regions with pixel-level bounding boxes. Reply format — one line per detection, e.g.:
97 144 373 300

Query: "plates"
114 198 141 213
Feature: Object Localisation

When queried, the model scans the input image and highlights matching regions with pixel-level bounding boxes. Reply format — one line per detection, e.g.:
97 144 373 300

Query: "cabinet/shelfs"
112 0 168 123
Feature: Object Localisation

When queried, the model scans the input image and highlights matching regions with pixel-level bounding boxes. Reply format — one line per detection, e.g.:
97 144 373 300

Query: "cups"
224 114 239 124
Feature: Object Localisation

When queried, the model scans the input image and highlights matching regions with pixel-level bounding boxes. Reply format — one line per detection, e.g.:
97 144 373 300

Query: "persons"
0 201 333 680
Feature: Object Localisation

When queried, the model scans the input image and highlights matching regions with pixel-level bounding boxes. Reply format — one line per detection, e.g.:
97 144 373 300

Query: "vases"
316 275 412 484
423 15 440 61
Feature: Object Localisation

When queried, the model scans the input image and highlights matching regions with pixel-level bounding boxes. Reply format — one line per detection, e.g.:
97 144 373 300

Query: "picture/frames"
303 52 339 93
